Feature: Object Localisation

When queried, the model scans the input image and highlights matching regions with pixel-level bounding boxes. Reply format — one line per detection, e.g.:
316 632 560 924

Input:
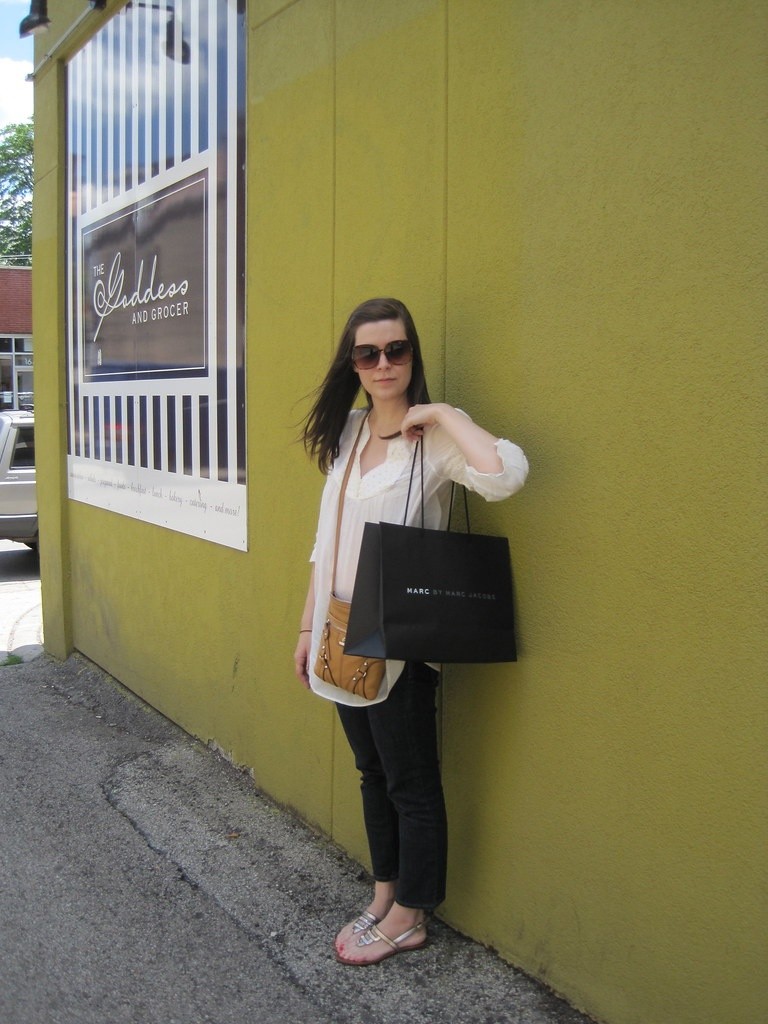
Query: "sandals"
337 917 433 967
334 907 396 951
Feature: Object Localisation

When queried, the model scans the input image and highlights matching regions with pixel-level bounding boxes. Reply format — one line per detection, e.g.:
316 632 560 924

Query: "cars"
0 407 45 557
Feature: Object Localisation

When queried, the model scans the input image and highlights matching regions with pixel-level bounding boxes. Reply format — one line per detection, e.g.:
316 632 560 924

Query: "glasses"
351 339 413 370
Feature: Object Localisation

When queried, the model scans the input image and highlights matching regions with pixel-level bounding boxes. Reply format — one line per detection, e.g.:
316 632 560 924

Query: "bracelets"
300 629 312 633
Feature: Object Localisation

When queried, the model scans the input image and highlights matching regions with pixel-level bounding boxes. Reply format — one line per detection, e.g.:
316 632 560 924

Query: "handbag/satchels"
314 593 386 699
343 427 518 665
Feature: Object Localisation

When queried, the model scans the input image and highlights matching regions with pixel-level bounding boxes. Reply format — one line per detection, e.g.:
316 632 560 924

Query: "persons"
292 296 531 967
